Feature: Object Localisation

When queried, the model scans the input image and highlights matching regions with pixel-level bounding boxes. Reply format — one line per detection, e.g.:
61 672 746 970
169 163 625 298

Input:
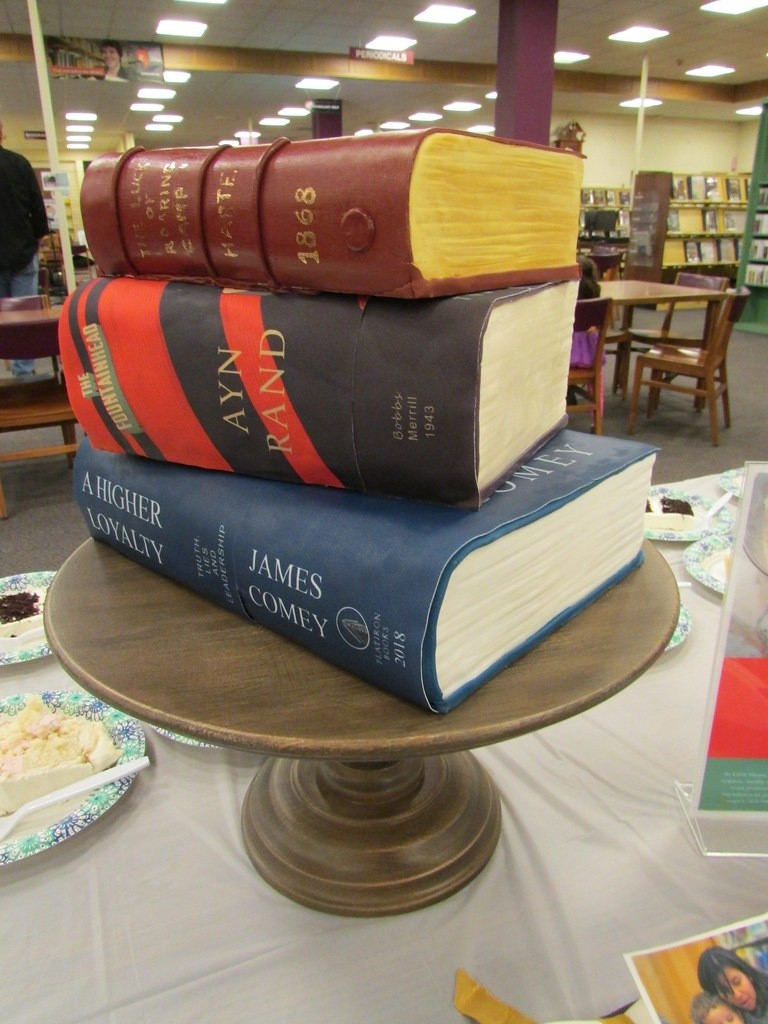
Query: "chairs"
621 272 728 410
628 287 750 447
0 318 81 518
565 297 612 436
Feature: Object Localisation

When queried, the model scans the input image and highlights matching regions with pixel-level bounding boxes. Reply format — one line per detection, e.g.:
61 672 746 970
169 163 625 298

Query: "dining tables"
598 280 727 409
0 469 767 1024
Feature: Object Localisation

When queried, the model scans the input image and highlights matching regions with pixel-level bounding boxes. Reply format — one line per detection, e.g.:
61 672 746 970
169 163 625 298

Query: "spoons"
0 756 151 842
682 488 734 536
0 627 45 651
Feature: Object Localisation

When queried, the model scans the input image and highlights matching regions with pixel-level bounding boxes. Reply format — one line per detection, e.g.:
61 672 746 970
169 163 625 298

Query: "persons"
99 42 138 82
0 122 50 379
566 254 608 407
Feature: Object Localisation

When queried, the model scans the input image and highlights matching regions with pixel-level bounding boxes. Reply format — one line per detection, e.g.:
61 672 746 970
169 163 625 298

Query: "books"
580 168 768 286
70 428 662 716
76 123 590 302
57 275 581 513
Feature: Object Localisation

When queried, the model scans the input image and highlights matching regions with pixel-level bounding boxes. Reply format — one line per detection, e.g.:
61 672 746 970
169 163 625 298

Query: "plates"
681 534 735 596
0 692 146 864
0 571 59 667
638 487 736 542
718 466 743 498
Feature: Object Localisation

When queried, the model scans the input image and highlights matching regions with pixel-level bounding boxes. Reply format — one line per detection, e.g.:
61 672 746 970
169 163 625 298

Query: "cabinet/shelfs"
733 98 768 334
624 171 753 310
578 188 634 279
